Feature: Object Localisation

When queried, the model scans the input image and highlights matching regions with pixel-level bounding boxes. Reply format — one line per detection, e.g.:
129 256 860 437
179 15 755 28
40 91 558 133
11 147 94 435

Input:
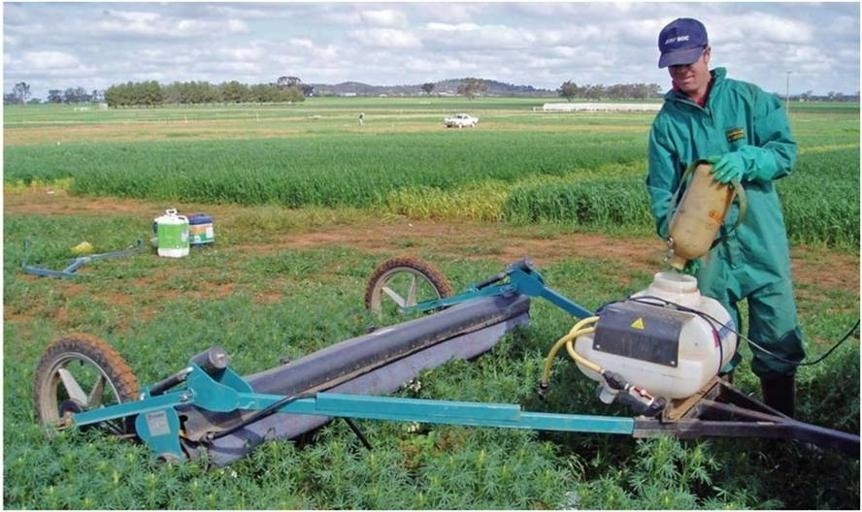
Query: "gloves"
707 146 777 189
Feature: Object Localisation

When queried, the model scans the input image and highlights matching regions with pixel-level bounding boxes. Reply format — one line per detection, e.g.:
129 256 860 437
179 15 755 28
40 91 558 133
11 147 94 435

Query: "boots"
761 379 822 461
700 371 733 443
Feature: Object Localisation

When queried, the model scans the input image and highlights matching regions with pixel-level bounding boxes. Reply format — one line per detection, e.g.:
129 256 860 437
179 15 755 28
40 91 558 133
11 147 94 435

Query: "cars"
444 113 477 128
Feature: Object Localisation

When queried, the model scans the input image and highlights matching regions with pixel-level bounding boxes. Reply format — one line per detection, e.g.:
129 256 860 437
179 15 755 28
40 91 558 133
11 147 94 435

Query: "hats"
658 18 708 68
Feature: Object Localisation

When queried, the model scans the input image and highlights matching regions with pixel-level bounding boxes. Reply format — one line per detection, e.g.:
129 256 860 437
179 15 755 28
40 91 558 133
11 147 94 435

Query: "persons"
645 17 806 471
